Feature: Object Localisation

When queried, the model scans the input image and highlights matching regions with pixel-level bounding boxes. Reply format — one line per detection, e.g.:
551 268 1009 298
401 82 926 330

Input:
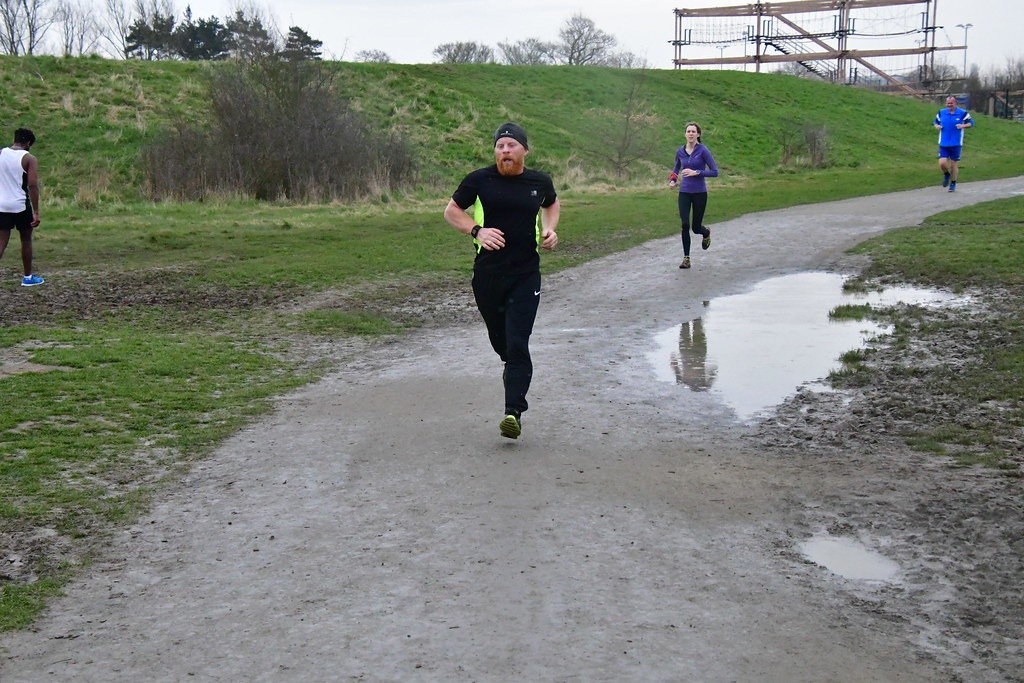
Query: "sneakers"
20 274 45 288
701 227 712 250
679 255 691 269
948 183 956 192
942 175 951 187
502 364 508 387
499 413 522 439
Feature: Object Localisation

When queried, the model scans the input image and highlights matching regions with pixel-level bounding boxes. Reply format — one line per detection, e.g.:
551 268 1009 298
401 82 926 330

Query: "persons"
0 127 44 286
670 121 718 269
934 96 974 192
444 123 560 443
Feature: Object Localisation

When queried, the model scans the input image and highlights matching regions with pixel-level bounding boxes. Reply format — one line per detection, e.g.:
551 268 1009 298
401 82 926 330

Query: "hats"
493 122 530 153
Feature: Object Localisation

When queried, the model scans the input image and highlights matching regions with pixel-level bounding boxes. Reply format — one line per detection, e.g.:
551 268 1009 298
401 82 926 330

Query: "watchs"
471 225 482 239
695 170 700 174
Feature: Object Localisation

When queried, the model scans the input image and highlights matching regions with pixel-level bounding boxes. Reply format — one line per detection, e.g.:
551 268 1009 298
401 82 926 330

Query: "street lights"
958 21 974 101
